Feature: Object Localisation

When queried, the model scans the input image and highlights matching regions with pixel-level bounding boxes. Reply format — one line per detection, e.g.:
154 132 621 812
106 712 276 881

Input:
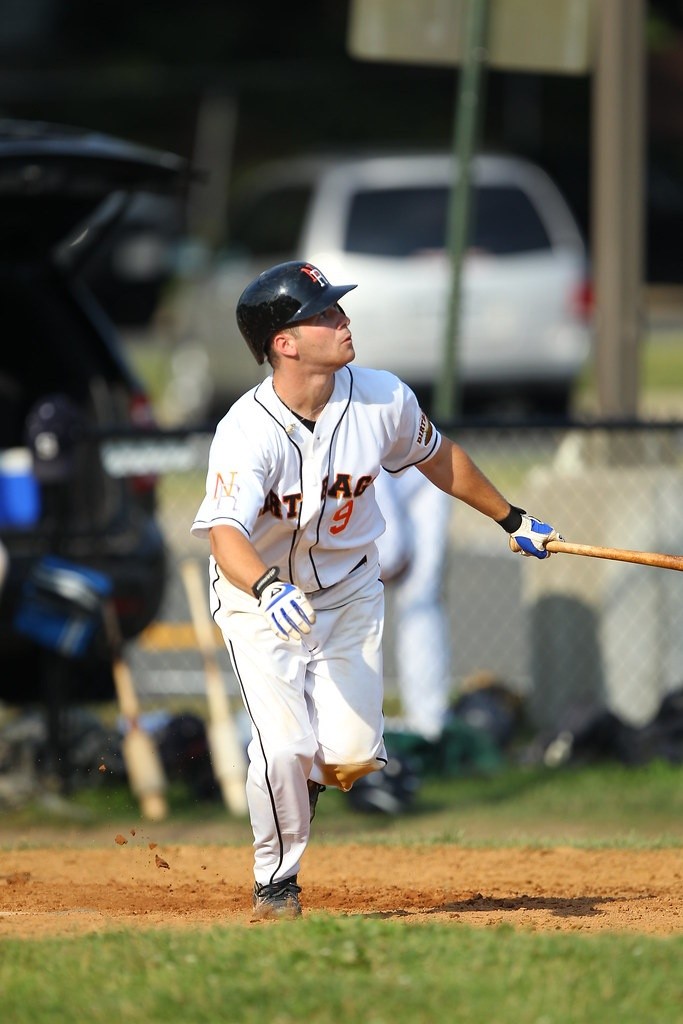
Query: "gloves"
251 566 317 641
494 503 566 559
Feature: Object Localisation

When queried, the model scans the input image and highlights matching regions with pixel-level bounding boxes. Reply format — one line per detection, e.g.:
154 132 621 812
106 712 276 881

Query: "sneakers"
253 873 302 922
306 779 322 823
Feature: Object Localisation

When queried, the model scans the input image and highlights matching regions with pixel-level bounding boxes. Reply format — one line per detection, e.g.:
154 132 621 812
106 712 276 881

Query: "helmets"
236 261 359 364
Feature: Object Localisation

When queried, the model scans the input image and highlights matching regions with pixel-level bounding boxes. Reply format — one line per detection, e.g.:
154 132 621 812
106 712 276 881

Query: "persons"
190 258 561 920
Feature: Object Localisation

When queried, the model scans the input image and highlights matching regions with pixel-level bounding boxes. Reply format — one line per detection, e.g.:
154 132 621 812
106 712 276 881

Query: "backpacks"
0 402 168 712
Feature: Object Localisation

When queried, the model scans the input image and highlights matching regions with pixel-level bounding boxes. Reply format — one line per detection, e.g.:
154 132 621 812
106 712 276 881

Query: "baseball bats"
509 534 683 574
99 597 168 821
177 561 251 816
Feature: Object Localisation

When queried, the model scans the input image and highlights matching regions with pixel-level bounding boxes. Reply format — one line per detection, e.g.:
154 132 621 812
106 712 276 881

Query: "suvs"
1 119 206 448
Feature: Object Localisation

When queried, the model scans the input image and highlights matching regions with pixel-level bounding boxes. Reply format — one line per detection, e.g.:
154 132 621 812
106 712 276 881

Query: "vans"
169 151 592 429
